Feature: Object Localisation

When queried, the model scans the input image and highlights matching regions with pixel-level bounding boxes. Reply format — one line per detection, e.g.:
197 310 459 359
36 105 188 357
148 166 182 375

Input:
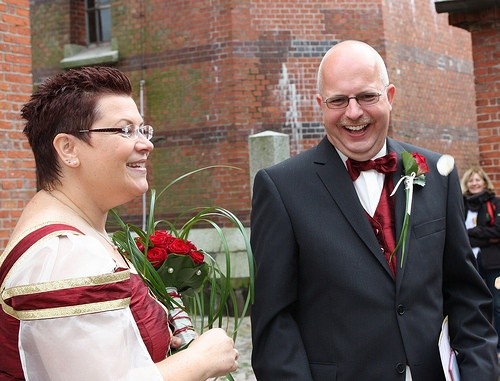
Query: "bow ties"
346 149 398 180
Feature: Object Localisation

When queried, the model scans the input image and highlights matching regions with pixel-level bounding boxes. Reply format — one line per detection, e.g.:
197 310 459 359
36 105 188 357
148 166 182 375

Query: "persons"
249 39 500 381
0 65 241 381
460 164 500 357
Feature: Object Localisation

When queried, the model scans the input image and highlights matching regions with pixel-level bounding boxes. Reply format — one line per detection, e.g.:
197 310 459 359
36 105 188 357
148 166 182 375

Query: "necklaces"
43 184 118 250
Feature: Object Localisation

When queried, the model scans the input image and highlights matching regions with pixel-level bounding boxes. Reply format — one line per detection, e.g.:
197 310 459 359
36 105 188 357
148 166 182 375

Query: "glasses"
321 89 385 110
67 124 154 142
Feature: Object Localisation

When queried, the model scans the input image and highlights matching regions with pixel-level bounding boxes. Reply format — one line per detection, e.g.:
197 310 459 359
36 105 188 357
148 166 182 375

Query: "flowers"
106 164 255 381
389 151 429 268
